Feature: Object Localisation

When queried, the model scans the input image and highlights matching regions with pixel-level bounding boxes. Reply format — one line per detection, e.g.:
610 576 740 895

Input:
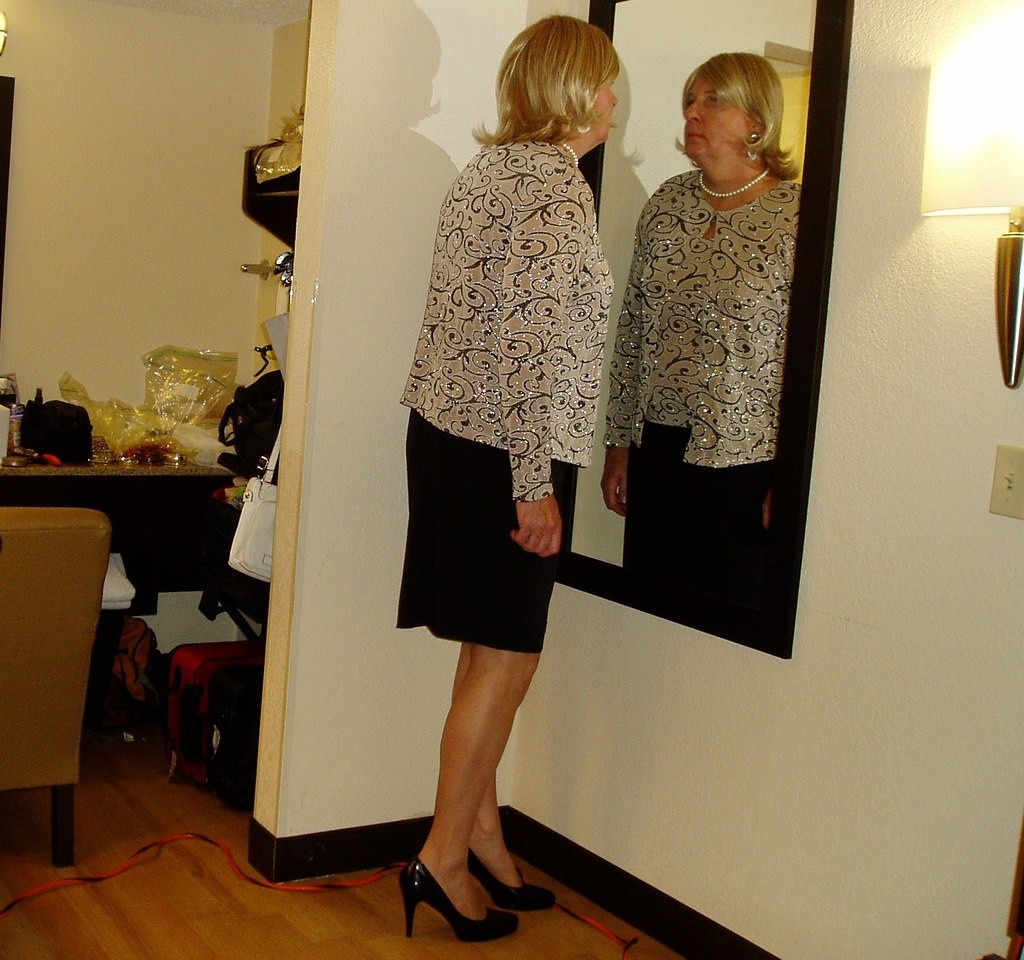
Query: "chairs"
0 506 113 867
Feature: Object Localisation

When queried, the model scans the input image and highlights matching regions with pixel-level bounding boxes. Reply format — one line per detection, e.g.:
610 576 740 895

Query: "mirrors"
556 0 856 660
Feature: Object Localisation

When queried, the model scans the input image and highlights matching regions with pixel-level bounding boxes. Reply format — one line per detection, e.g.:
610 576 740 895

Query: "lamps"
920 40 1024 389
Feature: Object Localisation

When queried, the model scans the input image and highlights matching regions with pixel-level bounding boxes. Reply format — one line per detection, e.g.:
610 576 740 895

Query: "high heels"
399 856 519 942
468 848 557 912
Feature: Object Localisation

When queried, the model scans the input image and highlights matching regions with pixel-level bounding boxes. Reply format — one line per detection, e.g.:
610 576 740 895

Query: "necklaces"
699 166 770 198
560 140 579 168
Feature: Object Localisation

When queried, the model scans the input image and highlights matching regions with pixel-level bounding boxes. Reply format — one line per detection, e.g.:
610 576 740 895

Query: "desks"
0 461 249 622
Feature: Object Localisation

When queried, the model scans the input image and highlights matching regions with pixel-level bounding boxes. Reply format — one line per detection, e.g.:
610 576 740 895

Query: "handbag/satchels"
218 368 283 476
228 477 277 584
97 620 264 813
19 400 92 467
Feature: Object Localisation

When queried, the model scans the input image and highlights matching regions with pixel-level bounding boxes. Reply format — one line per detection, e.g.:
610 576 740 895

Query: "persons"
603 52 801 622
396 15 620 943
